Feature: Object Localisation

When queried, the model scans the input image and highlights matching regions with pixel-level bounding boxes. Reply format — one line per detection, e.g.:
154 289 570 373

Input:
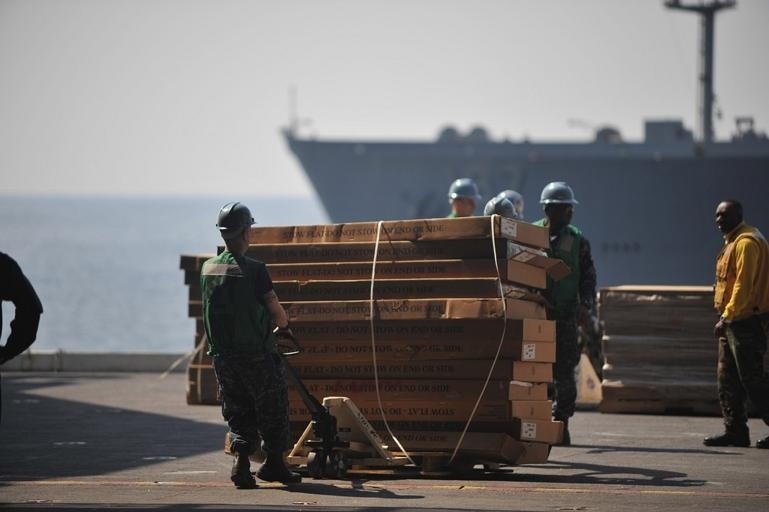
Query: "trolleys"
272 319 414 481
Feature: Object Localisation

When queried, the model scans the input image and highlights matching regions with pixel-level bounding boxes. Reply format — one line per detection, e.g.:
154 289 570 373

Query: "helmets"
484 196 518 218
539 180 579 205
215 201 257 230
448 177 482 201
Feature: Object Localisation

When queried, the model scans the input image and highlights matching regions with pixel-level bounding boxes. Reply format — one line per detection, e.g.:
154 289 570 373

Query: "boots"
552 416 570 446
255 455 302 485
231 453 256 488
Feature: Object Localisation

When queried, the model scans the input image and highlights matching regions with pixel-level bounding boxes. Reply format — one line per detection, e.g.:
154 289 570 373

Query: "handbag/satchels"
575 354 605 410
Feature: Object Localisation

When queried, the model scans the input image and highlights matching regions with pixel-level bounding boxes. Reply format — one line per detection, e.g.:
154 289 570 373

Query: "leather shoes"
703 427 750 447
755 436 768 448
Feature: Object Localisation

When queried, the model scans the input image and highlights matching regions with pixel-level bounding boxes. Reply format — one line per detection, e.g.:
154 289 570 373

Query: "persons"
0 252 45 371
485 197 521 258
198 199 304 488
703 195 769 449
527 180 599 447
444 176 482 220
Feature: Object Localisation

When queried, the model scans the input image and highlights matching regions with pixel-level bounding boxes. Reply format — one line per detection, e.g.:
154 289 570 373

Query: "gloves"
272 325 294 340
